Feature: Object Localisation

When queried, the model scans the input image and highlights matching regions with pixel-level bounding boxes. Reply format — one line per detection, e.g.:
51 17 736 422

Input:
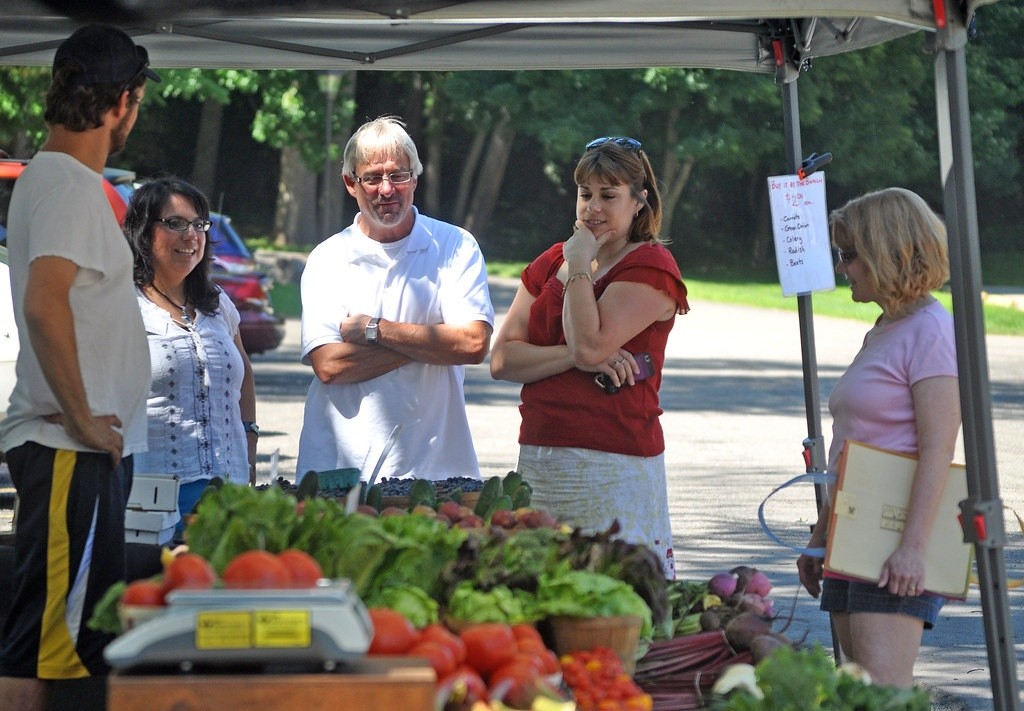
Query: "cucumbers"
294 469 533 523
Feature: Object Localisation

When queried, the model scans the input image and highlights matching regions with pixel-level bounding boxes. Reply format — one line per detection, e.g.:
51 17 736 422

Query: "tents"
0 0 1021 711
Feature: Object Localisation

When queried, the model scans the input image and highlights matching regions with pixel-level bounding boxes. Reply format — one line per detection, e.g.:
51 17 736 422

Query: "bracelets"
243 422 259 437
562 272 596 295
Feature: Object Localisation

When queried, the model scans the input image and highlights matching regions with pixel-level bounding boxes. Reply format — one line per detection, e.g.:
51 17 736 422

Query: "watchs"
364 318 381 344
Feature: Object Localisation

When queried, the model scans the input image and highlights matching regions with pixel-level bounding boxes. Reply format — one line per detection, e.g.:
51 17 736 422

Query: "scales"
104 576 377 673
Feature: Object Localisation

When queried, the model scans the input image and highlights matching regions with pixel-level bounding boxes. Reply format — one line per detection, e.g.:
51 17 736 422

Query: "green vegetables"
180 479 672 658
719 641 929 711
88 572 165 633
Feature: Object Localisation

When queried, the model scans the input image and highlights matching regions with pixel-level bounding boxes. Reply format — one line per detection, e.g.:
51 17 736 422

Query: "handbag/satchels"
758 440 974 602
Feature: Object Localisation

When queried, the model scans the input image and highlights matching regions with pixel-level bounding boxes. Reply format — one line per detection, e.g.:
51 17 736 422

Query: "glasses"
151 217 213 232
585 137 647 181
352 170 414 183
123 46 150 94
839 252 858 265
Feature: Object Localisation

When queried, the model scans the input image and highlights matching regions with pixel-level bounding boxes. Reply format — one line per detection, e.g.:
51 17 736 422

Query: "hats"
52 24 161 83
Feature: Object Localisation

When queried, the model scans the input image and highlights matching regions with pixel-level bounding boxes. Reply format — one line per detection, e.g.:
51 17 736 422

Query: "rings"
908 586 915 590
612 361 619 368
620 359 625 363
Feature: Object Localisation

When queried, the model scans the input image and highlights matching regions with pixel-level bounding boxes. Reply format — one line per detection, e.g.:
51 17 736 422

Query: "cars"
0 151 286 357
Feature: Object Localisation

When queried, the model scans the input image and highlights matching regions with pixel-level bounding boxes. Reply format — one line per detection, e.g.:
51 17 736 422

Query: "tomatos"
118 546 644 711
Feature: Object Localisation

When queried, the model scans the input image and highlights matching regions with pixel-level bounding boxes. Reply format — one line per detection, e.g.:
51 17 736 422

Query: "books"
820 440 974 602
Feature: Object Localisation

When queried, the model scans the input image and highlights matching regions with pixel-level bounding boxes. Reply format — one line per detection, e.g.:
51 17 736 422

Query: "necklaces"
152 283 191 321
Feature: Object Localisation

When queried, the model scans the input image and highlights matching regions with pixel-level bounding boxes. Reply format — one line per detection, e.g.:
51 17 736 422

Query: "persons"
122 178 259 579
294 118 495 486
0 24 162 711
490 137 690 580
797 188 962 691
